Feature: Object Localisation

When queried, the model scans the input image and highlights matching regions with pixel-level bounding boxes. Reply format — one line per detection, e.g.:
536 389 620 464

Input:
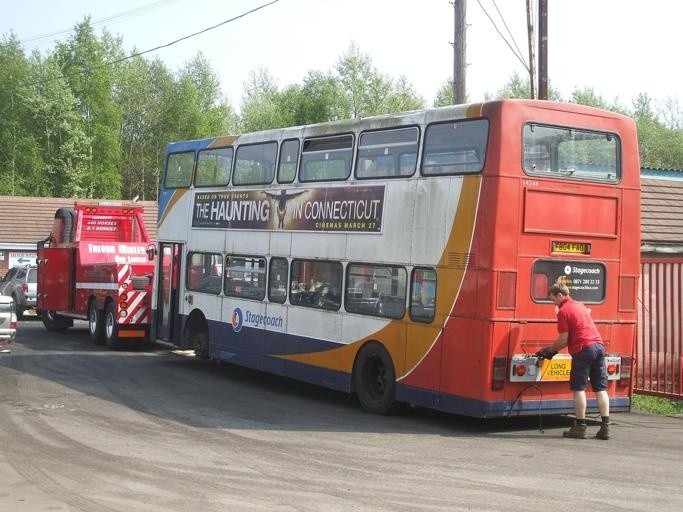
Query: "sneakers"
596 423 611 440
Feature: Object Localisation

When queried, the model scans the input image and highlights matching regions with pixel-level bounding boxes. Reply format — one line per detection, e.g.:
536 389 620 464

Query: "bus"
146 99 641 421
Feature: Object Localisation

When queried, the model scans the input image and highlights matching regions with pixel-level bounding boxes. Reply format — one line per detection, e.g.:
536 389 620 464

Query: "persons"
535 283 611 440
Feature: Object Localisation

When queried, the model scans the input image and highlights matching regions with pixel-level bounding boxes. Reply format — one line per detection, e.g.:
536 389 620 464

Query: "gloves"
532 347 558 361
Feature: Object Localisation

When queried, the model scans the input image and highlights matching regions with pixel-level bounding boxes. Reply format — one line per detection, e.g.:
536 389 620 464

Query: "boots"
563 420 588 439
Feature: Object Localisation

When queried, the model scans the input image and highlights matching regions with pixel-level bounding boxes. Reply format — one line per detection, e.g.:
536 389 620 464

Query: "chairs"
380 302 406 316
410 301 427 317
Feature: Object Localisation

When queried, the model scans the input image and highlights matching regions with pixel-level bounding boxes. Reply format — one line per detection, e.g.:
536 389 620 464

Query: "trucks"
36 201 177 342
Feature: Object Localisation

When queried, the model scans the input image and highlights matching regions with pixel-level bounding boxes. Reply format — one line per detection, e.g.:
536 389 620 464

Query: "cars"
0 265 37 347
